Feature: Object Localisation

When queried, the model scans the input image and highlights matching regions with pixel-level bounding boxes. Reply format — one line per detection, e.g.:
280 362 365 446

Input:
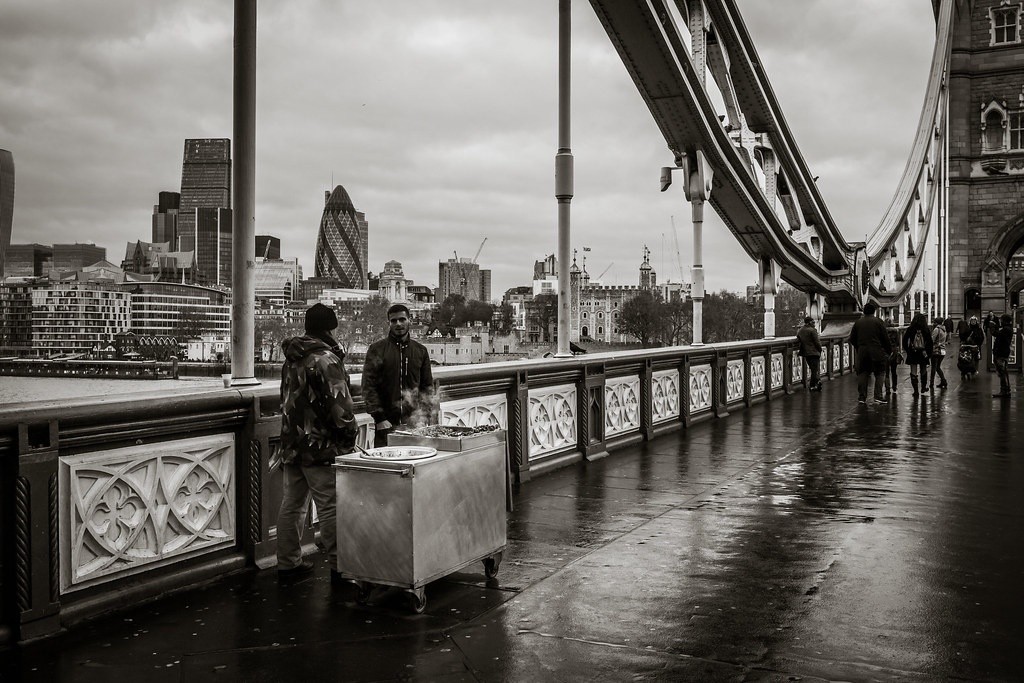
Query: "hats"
998 314 1013 323
934 316 943 324
305 302 338 330
805 316 813 323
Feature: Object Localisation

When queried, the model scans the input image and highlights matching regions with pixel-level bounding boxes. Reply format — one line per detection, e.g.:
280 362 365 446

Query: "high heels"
929 379 934 387
936 379 947 387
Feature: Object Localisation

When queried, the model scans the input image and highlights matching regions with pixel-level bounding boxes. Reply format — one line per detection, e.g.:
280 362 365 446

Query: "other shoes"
811 386 818 390
992 391 1011 397
278 560 314 576
874 397 887 403
858 398 866 404
975 370 978 375
818 381 823 391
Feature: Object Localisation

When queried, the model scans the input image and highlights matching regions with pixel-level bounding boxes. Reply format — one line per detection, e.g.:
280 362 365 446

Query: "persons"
797 316 823 391
944 315 954 344
361 304 434 448
903 313 933 394
991 314 1014 397
277 303 358 593
884 316 899 393
851 303 891 403
956 316 985 375
983 311 999 329
930 318 948 387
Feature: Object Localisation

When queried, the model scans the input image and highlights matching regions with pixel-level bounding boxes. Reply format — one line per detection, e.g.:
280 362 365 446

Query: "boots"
910 373 919 395
920 371 929 393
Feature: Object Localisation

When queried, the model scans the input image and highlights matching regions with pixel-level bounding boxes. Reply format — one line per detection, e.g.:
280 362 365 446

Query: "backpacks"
910 325 925 349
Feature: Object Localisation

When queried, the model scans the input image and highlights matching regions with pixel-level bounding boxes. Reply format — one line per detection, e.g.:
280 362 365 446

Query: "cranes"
450 236 490 299
593 261 615 281
668 215 688 293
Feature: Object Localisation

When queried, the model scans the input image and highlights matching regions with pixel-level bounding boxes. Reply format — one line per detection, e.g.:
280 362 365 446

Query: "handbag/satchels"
933 344 940 355
896 351 904 364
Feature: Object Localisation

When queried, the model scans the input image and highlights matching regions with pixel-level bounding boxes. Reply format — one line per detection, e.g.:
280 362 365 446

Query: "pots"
361 446 438 460
